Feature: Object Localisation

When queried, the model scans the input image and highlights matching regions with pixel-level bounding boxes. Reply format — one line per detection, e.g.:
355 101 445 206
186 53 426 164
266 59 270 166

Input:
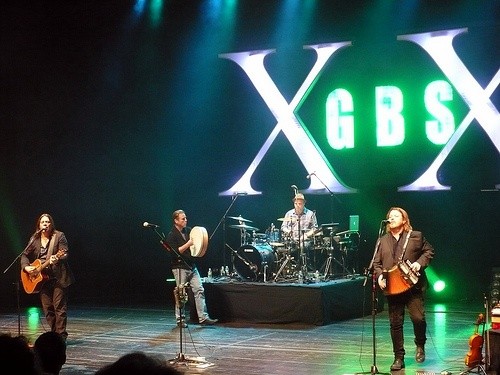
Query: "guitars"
21 248 67 294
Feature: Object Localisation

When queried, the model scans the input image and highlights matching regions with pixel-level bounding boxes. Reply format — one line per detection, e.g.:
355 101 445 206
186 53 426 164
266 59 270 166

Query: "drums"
253 229 279 244
189 226 208 257
234 243 277 281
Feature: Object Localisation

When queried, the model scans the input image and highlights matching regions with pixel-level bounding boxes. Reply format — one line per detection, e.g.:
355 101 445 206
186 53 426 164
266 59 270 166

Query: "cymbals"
294 228 312 231
228 224 260 232
227 216 252 222
277 218 299 221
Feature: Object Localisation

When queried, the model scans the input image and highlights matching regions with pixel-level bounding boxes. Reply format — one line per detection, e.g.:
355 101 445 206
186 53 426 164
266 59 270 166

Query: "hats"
292 194 306 202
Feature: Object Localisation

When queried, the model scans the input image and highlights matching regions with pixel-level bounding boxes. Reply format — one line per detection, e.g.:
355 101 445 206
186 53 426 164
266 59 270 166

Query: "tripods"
355 224 392 375
153 228 210 367
270 175 354 284
460 315 487 375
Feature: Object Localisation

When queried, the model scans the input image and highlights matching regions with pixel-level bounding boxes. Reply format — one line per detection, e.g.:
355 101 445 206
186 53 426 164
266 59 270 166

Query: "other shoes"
199 317 218 326
177 320 188 328
390 359 405 370
415 345 425 362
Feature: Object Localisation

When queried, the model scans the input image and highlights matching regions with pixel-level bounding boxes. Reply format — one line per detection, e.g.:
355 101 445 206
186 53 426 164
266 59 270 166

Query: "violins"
464 313 483 365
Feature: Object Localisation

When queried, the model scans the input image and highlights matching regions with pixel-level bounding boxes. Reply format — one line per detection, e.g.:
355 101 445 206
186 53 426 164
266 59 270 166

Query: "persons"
372 206 433 370
164 210 219 328
20 212 73 344
0 330 184 375
280 192 318 250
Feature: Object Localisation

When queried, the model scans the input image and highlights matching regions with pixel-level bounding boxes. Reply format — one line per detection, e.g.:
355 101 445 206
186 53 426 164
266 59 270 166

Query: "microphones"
306 171 316 179
36 226 47 234
143 222 161 228
382 220 391 223
